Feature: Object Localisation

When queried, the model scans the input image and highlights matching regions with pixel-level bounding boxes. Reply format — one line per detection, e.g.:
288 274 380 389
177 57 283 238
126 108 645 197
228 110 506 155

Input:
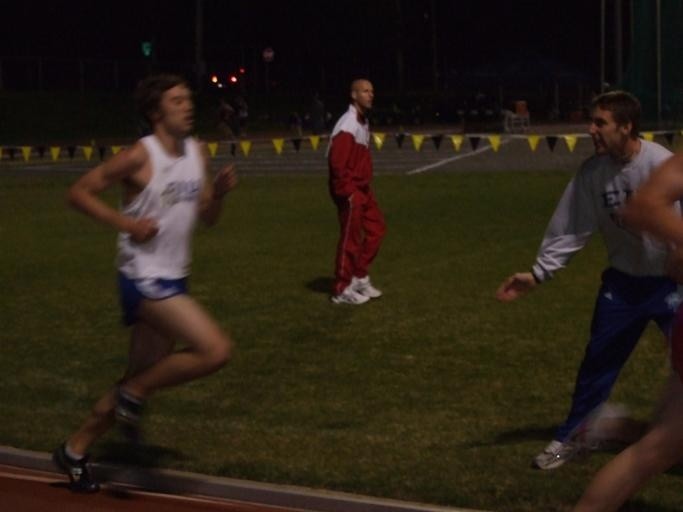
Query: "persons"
568 146 681 511
324 78 383 308
497 89 681 470
50 73 239 495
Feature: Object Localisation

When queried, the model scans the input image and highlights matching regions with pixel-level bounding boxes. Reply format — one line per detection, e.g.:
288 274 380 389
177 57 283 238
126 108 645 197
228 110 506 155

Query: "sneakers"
536 440 573 470
331 275 382 304
53 440 99 492
